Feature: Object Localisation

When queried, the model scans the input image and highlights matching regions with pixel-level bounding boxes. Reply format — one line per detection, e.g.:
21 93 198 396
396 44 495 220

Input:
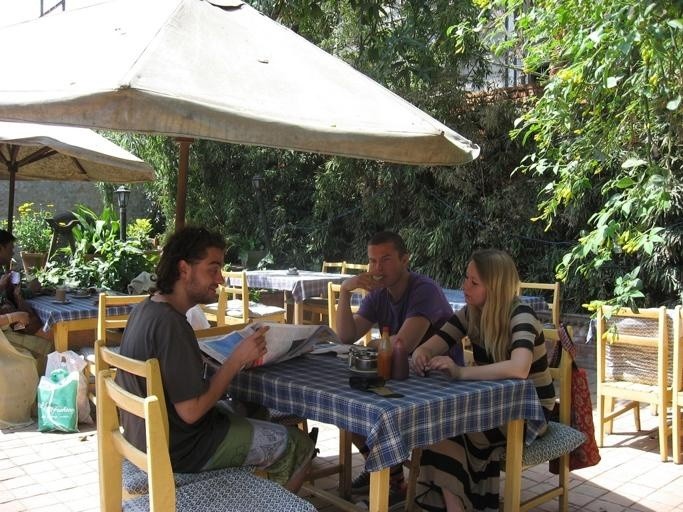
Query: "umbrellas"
5 1 483 229
0 120 157 233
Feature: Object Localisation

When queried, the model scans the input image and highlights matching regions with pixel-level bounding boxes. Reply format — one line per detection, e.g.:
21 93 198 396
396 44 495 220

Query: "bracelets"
5 312 13 325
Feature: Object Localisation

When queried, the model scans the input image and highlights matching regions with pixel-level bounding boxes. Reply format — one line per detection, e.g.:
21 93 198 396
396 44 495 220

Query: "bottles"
377 326 394 381
391 338 410 381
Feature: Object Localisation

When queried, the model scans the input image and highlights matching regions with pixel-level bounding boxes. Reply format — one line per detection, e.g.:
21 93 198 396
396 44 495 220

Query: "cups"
9 271 20 284
54 289 65 301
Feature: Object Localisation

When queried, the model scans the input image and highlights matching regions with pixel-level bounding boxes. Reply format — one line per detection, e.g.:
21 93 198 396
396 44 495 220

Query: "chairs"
595 302 681 465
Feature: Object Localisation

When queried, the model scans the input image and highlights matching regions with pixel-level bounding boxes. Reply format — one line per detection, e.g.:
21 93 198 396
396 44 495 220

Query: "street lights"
114 185 131 243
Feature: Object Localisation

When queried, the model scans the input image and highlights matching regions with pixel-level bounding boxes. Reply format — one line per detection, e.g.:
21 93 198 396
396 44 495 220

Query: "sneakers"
352 466 403 492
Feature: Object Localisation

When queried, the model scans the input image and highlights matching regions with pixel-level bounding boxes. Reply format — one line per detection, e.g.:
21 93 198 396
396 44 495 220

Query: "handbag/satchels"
542 323 601 474
37 350 95 432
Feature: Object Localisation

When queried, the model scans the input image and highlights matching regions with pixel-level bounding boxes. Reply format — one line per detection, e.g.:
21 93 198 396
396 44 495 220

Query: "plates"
71 295 90 299
50 299 70 303
349 364 378 374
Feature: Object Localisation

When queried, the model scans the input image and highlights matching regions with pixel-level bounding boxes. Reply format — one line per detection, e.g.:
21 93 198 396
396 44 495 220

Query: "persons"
333 232 464 511
0 311 55 428
0 230 45 336
110 225 314 494
410 246 558 511
